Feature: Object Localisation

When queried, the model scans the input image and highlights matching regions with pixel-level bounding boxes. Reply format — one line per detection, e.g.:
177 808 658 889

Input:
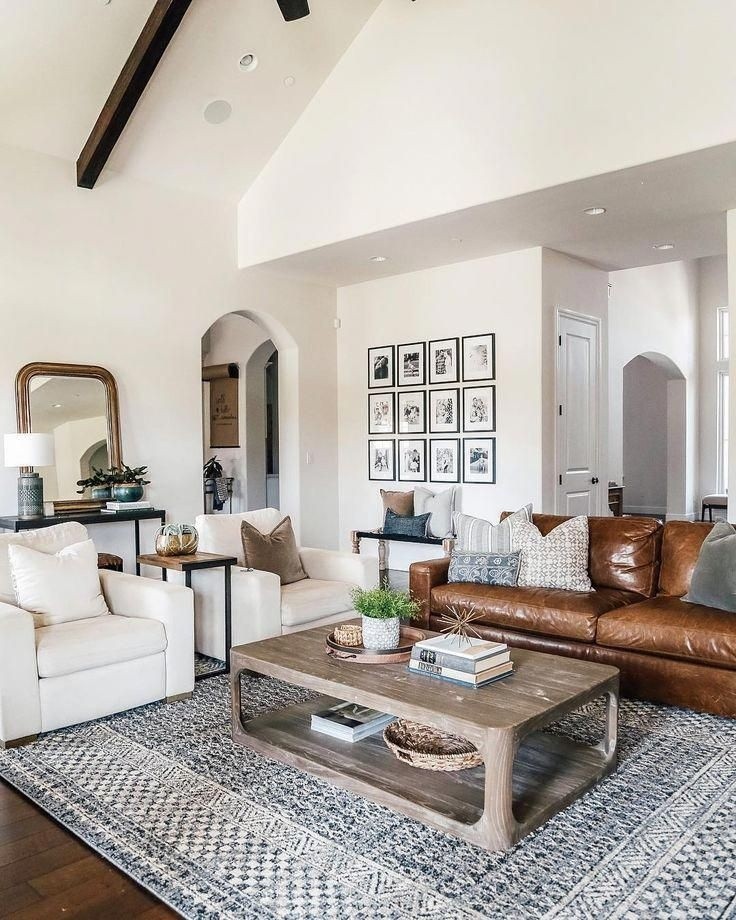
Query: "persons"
437 404 448 424
471 397 488 423
374 452 388 471
374 356 389 380
435 350 451 374
407 356 416 375
374 401 389 426
404 449 420 472
404 403 419 424
473 453 485 474
437 449 452 473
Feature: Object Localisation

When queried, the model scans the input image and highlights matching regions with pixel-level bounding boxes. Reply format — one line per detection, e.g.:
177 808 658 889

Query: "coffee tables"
227 618 616 852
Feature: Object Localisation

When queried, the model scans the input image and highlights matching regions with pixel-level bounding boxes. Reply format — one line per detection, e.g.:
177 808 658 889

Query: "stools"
97 551 123 572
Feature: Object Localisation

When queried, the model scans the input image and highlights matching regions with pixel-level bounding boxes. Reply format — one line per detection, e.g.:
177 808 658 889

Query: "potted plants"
351 579 424 650
76 466 110 500
110 464 149 500
204 456 222 495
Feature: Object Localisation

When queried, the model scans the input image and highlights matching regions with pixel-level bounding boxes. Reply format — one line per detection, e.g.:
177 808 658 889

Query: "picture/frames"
367 345 496 486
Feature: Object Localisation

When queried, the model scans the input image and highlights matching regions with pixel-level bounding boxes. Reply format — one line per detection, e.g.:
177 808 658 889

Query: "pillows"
680 515 736 617
237 515 310 589
415 486 456 539
383 511 430 537
449 504 534 552
380 491 413 516
8 542 116 625
509 517 597 593
449 550 522 590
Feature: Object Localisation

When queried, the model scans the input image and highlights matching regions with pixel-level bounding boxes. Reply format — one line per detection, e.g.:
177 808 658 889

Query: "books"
407 632 513 690
310 701 398 743
101 502 154 514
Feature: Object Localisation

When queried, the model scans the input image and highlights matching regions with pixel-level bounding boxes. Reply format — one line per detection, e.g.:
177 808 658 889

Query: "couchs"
0 521 195 750
409 511 736 722
166 507 381 665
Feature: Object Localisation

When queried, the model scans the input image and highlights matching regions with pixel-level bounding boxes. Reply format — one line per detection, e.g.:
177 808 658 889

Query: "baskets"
382 717 484 772
333 624 363 647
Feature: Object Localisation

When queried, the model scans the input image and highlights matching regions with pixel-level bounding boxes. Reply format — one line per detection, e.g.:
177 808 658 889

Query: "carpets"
4 658 736 920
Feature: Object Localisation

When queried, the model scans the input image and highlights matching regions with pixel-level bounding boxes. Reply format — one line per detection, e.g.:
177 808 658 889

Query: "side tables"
136 552 237 680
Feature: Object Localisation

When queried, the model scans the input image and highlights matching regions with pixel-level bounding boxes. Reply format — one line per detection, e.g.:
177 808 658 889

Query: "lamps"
4 432 53 521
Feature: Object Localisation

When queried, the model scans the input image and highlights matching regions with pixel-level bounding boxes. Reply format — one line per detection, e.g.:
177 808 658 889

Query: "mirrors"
15 364 123 510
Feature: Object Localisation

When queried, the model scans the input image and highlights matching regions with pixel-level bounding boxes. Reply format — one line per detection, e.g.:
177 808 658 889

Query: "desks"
351 527 456 590
702 494 728 523
3 506 169 583
609 486 626 517
